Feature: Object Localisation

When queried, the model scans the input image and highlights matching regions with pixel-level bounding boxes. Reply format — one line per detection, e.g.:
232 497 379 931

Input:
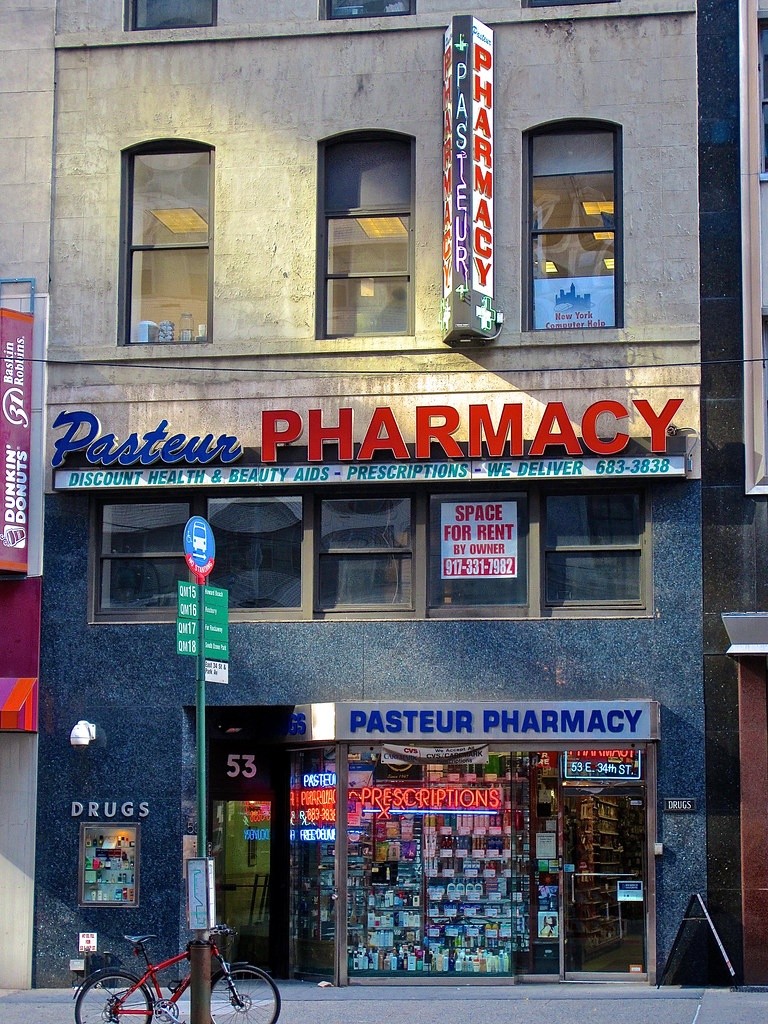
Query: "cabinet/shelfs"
298 775 644 975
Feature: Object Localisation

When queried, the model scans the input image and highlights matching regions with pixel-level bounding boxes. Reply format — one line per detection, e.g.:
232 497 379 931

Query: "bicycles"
73 923 281 1024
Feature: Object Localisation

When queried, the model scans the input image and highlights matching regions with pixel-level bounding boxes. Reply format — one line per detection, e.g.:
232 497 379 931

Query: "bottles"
348 934 510 973
85 834 134 901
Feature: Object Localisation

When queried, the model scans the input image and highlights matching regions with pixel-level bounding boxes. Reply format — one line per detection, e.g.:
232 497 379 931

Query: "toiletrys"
342 807 517 976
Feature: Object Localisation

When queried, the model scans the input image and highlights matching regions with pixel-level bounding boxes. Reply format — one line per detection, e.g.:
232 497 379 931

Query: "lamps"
70 720 96 745
